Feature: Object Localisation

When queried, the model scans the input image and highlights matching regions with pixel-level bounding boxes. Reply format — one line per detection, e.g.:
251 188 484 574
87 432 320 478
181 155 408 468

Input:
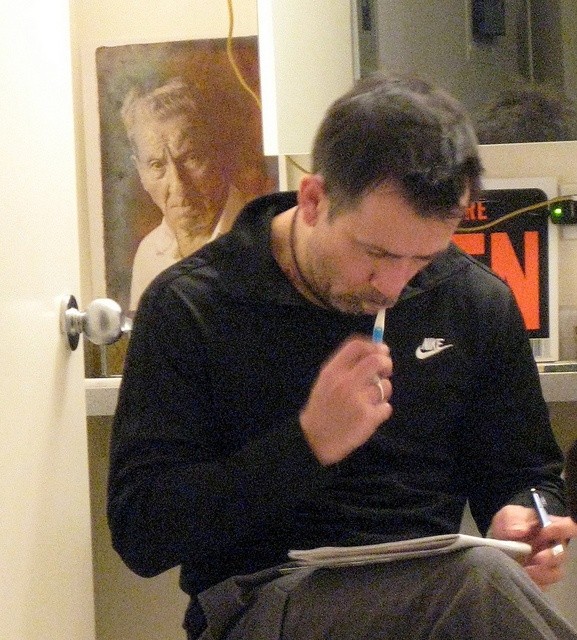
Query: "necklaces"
289 203 327 310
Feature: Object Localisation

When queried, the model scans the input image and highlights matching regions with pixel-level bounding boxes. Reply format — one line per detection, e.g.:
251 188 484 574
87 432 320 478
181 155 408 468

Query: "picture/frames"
85 33 264 379
444 177 558 364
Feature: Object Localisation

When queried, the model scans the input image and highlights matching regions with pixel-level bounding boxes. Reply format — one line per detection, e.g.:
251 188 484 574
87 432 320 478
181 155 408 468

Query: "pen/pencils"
371 309 385 341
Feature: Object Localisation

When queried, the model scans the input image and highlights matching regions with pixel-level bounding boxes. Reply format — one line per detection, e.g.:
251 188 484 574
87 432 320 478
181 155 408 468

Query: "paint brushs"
529 488 563 556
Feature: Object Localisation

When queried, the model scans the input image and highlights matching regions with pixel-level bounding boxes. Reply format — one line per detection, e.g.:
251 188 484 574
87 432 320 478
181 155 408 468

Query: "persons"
107 73 575 638
119 81 250 324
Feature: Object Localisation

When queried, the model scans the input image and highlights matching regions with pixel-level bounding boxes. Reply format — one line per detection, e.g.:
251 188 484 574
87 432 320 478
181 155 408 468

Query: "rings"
373 378 384 401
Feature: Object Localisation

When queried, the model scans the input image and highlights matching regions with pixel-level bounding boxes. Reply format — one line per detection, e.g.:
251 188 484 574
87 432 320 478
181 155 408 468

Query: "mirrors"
349 1 577 145
